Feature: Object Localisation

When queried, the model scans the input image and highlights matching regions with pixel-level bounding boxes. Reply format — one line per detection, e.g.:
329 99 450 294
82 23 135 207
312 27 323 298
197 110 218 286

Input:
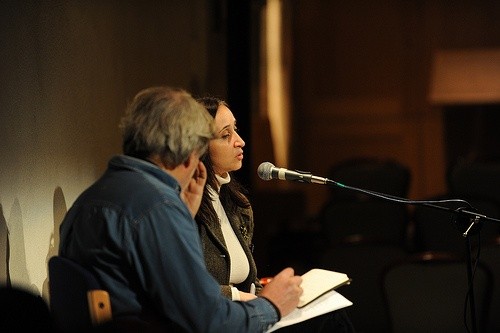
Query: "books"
296 268 352 308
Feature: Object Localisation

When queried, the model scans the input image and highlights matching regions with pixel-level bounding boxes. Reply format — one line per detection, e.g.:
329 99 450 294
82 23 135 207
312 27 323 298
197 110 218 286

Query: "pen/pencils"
258 277 273 284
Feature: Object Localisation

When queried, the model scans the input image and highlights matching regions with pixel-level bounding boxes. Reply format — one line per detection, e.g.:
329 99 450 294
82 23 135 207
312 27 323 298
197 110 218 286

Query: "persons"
58 86 301 333
195 96 263 302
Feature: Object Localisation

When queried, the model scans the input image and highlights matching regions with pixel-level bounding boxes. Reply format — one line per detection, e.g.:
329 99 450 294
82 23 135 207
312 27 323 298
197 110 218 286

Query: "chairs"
439 101 500 225
242 157 418 332
47 255 115 333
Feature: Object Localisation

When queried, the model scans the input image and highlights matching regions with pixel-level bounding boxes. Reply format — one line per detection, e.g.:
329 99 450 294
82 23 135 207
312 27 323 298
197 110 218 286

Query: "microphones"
257 162 336 185
